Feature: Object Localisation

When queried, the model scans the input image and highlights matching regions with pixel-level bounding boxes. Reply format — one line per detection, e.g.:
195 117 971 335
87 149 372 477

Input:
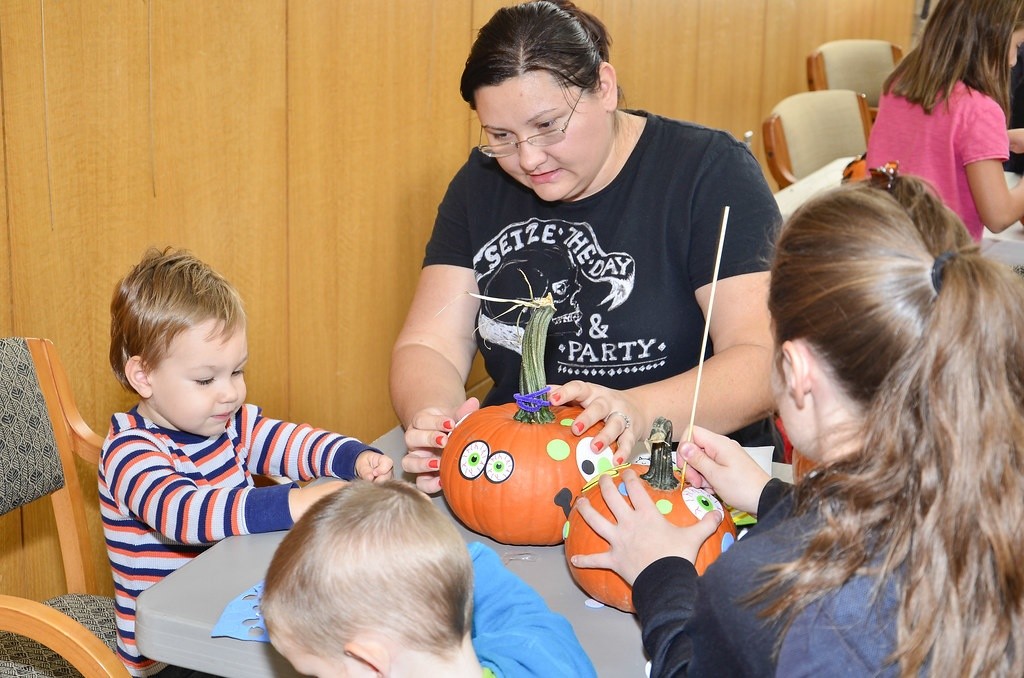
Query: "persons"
256 475 598 677
93 243 396 678
571 167 1023 678
865 1 1024 246
391 0 785 496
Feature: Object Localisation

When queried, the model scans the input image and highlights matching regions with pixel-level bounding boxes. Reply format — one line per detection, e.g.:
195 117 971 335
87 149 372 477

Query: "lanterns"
841 152 866 184
440 401 616 546
561 463 737 615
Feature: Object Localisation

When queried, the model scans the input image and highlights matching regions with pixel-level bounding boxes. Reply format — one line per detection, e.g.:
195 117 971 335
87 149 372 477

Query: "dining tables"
770 153 1023 274
135 403 799 678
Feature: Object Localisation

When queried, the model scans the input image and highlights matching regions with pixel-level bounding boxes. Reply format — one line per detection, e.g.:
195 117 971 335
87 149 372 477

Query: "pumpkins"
438 292 738 614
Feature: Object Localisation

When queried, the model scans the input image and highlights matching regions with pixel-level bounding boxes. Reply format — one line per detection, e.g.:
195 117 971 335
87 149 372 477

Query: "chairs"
760 38 909 192
0 334 205 677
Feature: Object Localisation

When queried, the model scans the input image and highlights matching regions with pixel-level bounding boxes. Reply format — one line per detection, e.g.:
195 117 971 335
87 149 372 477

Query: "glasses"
478 84 585 156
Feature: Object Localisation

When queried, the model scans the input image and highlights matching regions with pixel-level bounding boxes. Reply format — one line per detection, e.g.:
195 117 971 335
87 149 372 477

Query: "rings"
607 411 630 429
